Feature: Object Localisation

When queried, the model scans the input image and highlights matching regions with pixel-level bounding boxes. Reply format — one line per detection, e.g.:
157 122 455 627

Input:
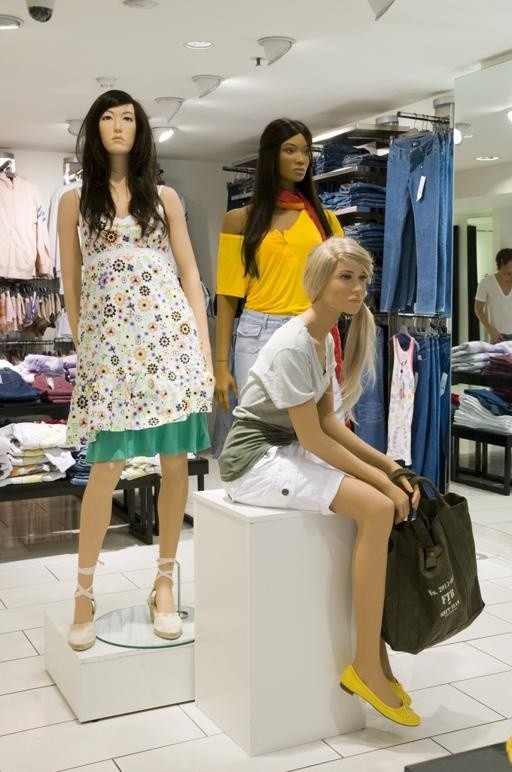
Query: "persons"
214 117 345 428
57 89 215 651
218 236 421 728
474 248 512 344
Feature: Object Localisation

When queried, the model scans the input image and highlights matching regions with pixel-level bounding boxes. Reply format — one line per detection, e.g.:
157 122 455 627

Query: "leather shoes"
339 664 421 728
389 676 411 706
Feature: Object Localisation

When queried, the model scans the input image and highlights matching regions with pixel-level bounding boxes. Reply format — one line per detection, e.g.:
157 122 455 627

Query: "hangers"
388 315 448 351
1 160 20 179
389 113 452 143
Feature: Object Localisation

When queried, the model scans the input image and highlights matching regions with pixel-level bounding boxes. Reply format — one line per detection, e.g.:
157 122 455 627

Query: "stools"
192 490 366 757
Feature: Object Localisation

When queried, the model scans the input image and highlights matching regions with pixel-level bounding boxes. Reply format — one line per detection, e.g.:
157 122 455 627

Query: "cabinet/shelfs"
451 370 512 496
232 124 412 405
1 384 210 545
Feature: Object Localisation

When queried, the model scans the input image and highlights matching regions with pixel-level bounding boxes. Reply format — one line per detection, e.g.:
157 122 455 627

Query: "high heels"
67 566 98 651
147 557 184 640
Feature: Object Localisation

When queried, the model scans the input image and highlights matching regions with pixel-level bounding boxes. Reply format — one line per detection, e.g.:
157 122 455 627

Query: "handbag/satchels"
381 468 486 656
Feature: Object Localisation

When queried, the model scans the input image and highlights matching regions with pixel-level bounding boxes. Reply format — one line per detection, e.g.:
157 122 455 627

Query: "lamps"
0 15 24 32
191 74 224 98
506 108 511 121
153 126 178 143
153 96 185 123
258 36 296 65
65 119 83 138
454 123 471 143
368 0 395 20
